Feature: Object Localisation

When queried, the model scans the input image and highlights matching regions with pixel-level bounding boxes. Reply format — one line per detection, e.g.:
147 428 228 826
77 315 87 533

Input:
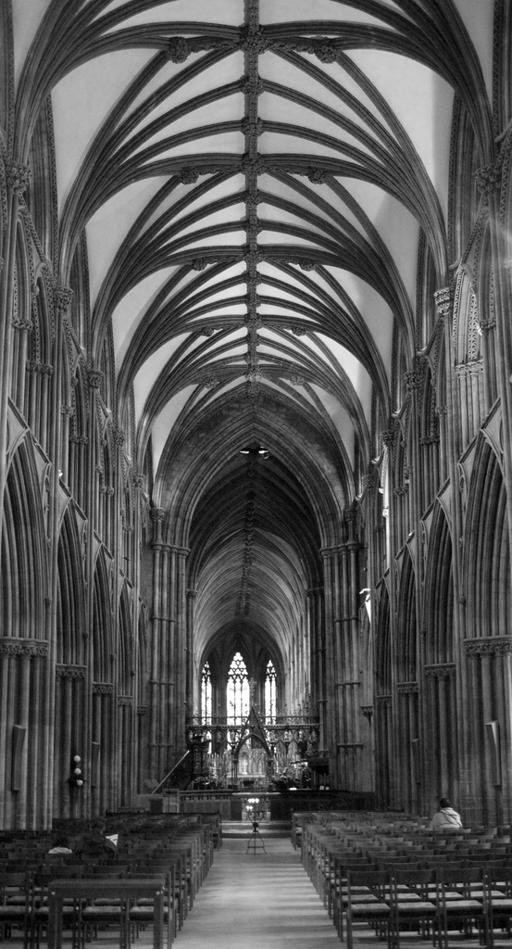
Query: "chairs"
1 810 224 949
291 807 511 949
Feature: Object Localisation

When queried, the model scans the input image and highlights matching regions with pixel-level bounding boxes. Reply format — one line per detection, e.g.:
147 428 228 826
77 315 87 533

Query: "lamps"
67 748 85 810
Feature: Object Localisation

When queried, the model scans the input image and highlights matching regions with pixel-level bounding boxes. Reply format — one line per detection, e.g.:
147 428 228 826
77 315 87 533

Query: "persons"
75 815 118 860
33 831 95 885
300 764 311 788
428 796 462 829
